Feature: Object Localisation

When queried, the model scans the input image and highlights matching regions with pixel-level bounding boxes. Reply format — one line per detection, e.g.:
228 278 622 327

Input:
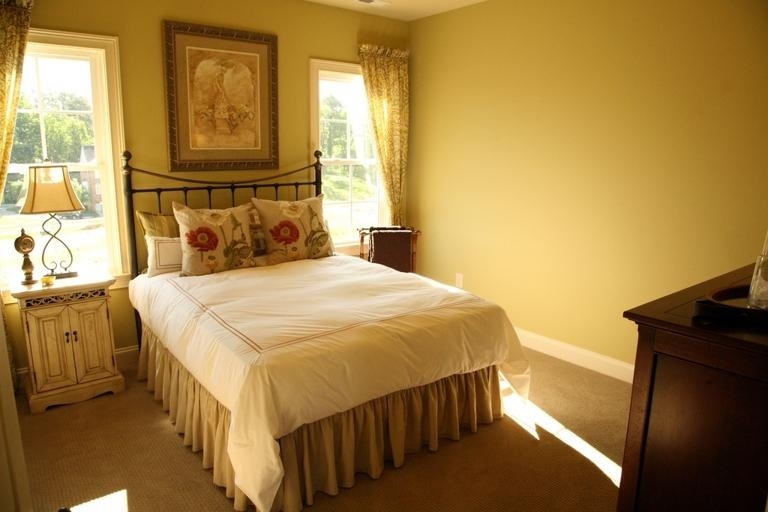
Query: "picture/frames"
162 18 281 173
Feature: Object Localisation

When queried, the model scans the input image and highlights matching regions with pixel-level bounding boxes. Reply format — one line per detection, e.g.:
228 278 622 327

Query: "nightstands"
11 273 125 416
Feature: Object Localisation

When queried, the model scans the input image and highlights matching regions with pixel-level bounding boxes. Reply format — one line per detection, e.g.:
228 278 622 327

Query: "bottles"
746 230 768 309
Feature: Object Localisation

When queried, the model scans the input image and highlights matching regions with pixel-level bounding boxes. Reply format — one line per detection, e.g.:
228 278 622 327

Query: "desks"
355 225 421 273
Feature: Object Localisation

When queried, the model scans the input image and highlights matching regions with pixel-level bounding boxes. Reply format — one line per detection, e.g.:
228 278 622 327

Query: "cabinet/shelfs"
614 262 767 511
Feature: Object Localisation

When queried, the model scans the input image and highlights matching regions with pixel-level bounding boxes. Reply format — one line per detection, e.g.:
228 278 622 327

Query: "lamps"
20 165 86 278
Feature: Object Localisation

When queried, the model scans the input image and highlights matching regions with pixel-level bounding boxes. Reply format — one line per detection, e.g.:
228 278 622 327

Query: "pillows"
136 192 334 277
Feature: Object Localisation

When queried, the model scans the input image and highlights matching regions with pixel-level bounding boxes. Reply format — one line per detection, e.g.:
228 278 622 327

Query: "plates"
704 281 768 318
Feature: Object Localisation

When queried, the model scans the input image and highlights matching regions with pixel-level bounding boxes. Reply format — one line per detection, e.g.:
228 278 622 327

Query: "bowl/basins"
40 276 56 286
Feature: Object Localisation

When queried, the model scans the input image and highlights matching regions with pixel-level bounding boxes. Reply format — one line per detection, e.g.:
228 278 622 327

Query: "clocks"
14 228 37 285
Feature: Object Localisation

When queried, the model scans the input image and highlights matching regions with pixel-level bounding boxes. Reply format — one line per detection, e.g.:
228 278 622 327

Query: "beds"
121 149 508 511
